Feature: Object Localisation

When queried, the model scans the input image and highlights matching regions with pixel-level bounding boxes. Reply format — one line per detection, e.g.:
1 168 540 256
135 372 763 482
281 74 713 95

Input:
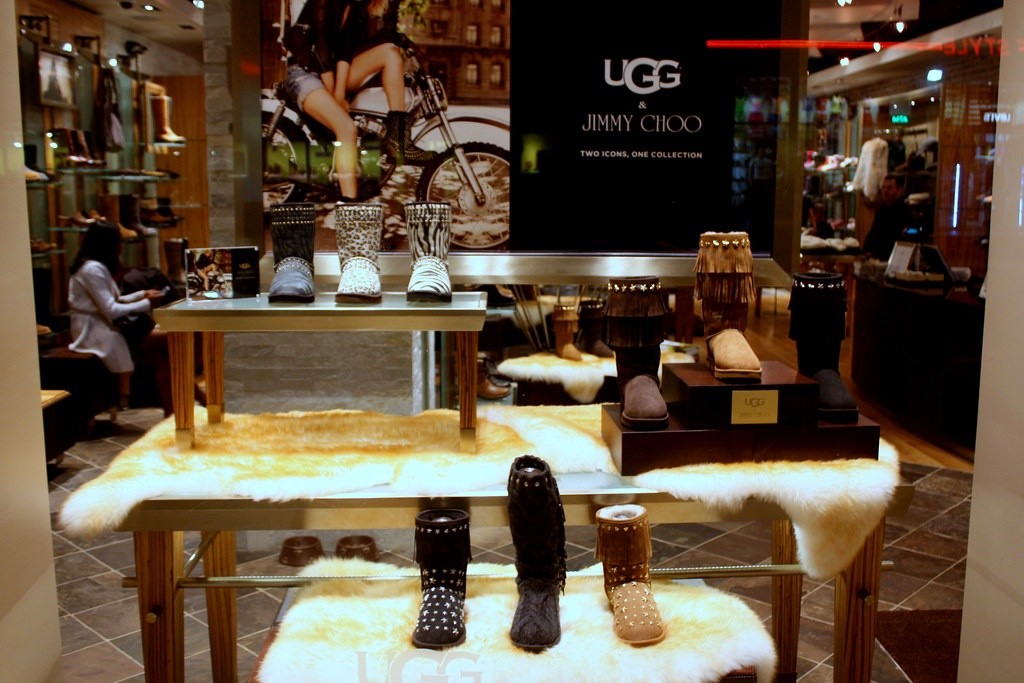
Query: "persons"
195 249 220 292
860 172 920 264
63 220 169 411
276 0 441 232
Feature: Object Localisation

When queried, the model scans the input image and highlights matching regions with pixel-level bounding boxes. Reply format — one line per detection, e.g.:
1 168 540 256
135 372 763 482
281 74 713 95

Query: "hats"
916 138 937 155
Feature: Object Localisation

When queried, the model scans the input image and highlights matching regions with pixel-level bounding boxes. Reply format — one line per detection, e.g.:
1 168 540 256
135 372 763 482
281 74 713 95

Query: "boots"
478 353 512 388
788 272 859 423
804 151 856 171
380 110 437 164
266 202 314 302
554 306 582 361
510 455 566 649
120 194 156 238
595 504 665 646
164 241 186 285
150 97 186 143
478 360 509 398
404 200 452 300
578 299 613 357
694 231 762 379
340 198 391 251
605 276 669 429
24 126 107 183
412 508 471 647
98 195 137 238
212 275 223 284
333 202 382 303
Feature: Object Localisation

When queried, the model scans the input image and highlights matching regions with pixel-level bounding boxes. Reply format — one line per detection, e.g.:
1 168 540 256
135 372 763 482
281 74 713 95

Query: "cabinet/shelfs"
60 292 899 683
24 126 186 258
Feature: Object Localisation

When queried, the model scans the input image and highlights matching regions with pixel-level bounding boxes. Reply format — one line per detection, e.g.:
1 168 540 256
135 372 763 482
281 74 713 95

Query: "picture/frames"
885 240 916 279
35 45 78 112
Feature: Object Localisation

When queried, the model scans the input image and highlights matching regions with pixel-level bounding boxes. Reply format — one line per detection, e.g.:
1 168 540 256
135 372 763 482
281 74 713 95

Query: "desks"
851 269 985 452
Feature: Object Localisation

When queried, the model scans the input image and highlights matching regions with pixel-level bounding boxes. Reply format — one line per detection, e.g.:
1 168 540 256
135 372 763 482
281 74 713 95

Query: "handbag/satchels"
113 299 155 348
100 68 125 154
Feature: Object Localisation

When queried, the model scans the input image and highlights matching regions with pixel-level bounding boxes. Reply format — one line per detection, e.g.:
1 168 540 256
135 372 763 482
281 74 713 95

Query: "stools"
41 347 120 429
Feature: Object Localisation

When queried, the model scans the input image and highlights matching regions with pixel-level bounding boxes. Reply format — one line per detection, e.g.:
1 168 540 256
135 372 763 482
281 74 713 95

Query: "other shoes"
158 197 172 216
59 212 96 227
472 285 516 307
464 284 512 297
31 240 49 253
36 323 50 334
83 210 107 222
181 238 188 270
37 240 57 250
139 198 169 222
801 234 859 248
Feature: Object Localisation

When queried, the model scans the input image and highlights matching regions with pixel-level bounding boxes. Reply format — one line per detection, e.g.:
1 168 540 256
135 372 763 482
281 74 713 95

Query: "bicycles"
258 49 512 252
